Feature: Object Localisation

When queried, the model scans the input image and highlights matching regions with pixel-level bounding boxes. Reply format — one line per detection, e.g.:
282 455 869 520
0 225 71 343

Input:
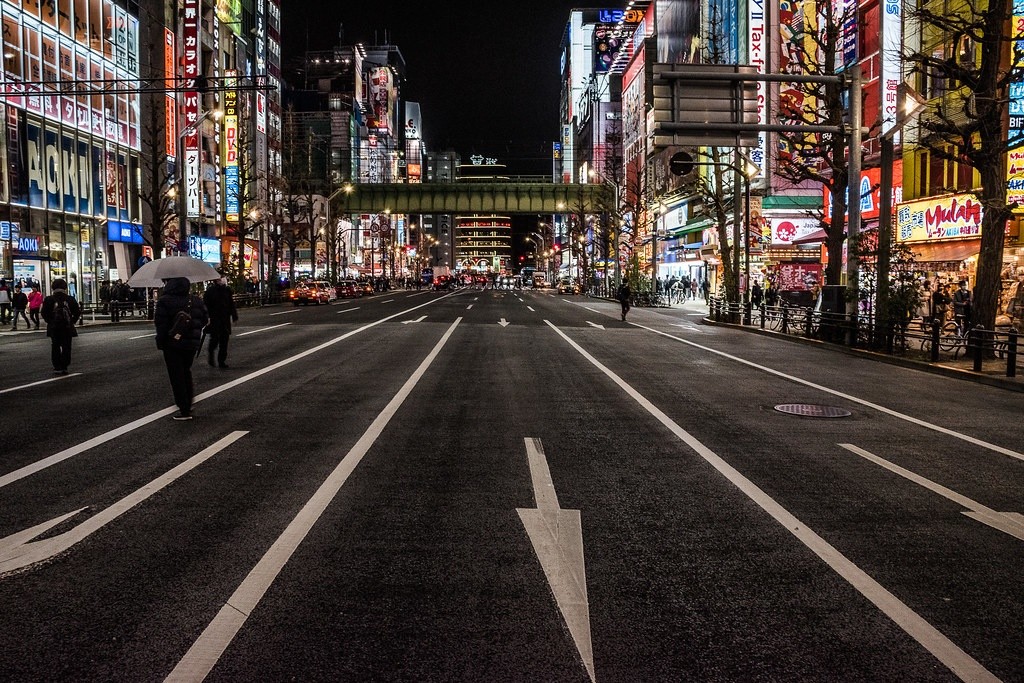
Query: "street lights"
370 208 390 279
531 232 545 251
324 184 352 274
399 223 415 279
539 222 557 288
524 237 539 269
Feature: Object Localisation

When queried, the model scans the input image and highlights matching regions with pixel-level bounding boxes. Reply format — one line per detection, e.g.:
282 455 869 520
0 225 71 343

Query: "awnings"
641 220 715 241
240 238 274 253
792 219 880 245
863 240 982 261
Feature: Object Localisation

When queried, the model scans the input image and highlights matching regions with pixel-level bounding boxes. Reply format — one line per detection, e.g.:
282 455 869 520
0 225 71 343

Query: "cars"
288 280 338 305
358 282 375 296
335 279 364 297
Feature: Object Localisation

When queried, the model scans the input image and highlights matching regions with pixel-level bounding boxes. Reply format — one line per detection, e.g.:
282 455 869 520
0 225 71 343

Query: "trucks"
531 271 545 288
419 266 457 291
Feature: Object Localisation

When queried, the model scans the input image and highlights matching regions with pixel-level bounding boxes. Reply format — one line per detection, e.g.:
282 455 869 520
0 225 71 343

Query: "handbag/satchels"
49 305 72 324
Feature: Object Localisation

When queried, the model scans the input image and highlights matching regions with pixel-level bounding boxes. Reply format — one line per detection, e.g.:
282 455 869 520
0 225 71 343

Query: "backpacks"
165 295 196 343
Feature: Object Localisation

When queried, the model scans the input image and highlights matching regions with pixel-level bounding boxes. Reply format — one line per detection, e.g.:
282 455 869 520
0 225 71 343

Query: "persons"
14 273 37 287
448 275 540 293
0 279 14 324
10 285 30 332
97 277 301 317
40 278 80 375
201 277 240 368
153 276 202 422
343 274 436 292
616 278 631 321
915 279 975 336
765 282 778 321
650 274 712 306
751 280 763 311
27 283 42 330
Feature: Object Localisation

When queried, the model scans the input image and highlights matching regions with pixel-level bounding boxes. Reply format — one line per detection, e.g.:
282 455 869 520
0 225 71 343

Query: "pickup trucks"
558 279 579 295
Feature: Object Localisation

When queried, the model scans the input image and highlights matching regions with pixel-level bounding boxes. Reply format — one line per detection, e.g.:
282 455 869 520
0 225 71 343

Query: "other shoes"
54 368 67 374
173 412 193 421
219 362 229 369
10 328 18 331
207 354 216 367
26 325 30 329
34 326 39 330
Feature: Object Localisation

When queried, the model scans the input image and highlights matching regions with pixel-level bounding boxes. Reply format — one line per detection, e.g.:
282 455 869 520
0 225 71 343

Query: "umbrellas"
125 255 222 289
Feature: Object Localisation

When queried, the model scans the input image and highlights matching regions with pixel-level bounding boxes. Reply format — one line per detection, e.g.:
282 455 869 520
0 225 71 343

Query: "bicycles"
769 294 818 333
673 288 687 305
940 313 972 352
